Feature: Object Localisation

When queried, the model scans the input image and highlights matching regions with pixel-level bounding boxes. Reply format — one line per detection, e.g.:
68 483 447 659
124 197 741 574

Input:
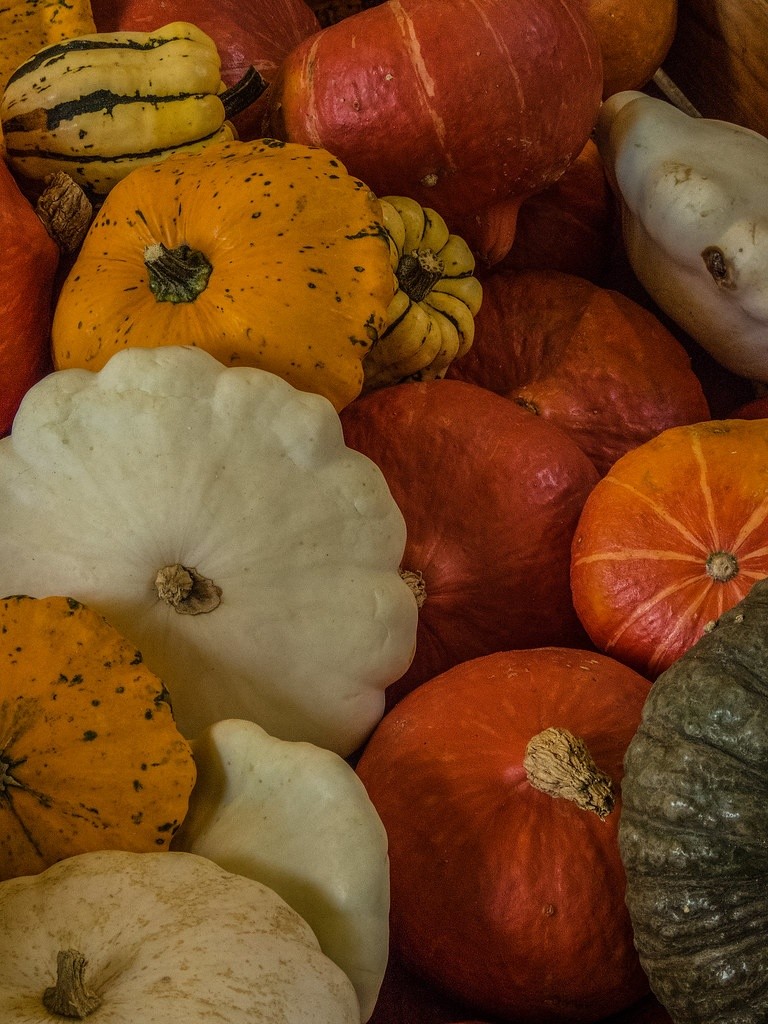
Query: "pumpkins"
0 0 768 1024
